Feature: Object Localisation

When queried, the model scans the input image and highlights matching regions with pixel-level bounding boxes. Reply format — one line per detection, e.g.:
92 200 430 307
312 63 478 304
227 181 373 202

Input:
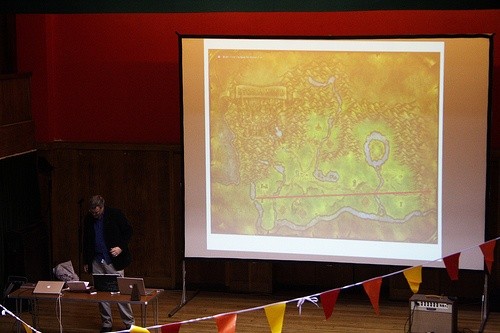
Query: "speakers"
408 294 458 333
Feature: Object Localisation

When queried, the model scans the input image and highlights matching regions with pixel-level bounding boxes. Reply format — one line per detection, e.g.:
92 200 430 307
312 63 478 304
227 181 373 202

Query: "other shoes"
100 327 112 333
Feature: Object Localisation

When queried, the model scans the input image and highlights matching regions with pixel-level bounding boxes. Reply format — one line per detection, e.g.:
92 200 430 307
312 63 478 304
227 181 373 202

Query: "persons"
82 195 135 333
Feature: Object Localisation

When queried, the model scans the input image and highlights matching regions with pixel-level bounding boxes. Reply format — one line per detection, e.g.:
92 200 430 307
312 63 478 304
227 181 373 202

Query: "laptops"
33 281 65 295
117 277 155 296
93 274 120 292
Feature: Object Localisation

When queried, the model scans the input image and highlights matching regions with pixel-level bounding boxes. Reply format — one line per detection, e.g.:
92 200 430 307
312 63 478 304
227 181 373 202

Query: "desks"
8 286 164 333
